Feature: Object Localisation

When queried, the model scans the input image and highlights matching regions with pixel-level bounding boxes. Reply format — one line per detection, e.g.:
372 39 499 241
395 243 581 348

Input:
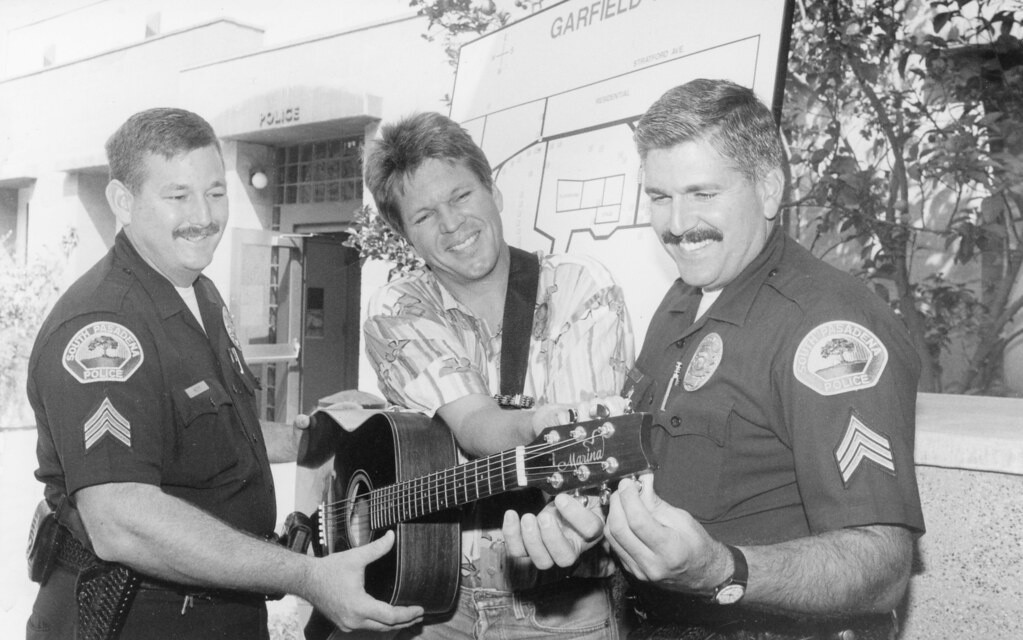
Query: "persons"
502 78 926 640
26 106 424 639
324 110 635 640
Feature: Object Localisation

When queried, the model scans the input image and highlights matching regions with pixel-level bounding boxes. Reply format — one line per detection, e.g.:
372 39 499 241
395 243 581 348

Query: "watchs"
701 543 748 606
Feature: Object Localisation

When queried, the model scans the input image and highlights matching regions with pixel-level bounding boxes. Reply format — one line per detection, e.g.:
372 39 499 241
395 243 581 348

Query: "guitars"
292 399 667 629
